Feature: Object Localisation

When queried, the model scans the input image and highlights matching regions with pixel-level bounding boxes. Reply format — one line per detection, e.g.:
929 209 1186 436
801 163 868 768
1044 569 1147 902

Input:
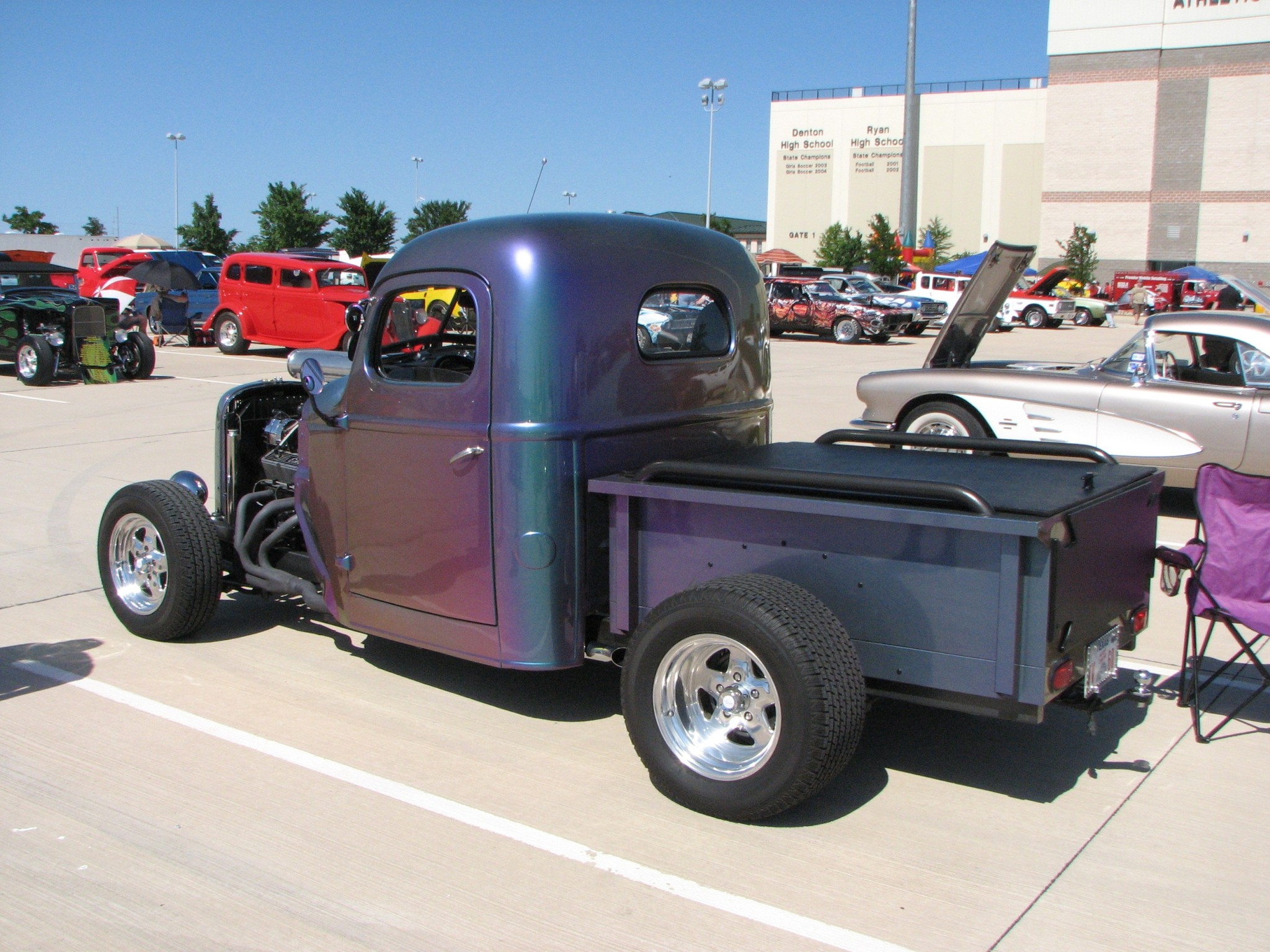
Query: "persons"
1088 279 1166 323
116 308 147 335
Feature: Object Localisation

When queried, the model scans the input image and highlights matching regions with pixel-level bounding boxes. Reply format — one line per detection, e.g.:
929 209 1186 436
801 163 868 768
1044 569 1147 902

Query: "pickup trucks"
96 212 1169 825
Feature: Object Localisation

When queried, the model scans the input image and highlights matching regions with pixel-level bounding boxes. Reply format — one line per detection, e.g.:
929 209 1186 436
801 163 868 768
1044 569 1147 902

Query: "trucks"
1111 270 1223 315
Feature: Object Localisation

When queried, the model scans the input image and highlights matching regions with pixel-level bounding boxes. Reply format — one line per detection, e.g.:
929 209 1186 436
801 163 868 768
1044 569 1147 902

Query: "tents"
1165 266 1223 283
851 251 1040 284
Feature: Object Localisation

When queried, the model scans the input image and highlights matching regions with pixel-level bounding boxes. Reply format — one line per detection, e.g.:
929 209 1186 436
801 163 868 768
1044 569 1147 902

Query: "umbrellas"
124 260 202 292
754 249 808 264
90 276 137 315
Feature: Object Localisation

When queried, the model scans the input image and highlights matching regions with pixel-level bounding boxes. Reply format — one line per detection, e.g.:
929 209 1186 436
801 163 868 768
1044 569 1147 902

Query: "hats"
1092 281 1096 284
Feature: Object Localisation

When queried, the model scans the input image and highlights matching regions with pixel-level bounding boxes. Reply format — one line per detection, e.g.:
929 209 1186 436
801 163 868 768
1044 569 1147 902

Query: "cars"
202 251 448 355
0 260 156 386
0 247 225 333
901 267 1113 330
850 240 1270 492
635 275 950 354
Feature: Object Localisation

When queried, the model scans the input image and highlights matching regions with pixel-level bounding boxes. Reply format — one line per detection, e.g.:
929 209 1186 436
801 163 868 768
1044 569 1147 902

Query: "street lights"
166 132 187 248
697 78 728 228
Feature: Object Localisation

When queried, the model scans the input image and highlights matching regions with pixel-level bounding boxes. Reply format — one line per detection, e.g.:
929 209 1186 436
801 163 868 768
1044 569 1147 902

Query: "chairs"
778 286 795 299
151 297 190 348
1155 460 1270 744
